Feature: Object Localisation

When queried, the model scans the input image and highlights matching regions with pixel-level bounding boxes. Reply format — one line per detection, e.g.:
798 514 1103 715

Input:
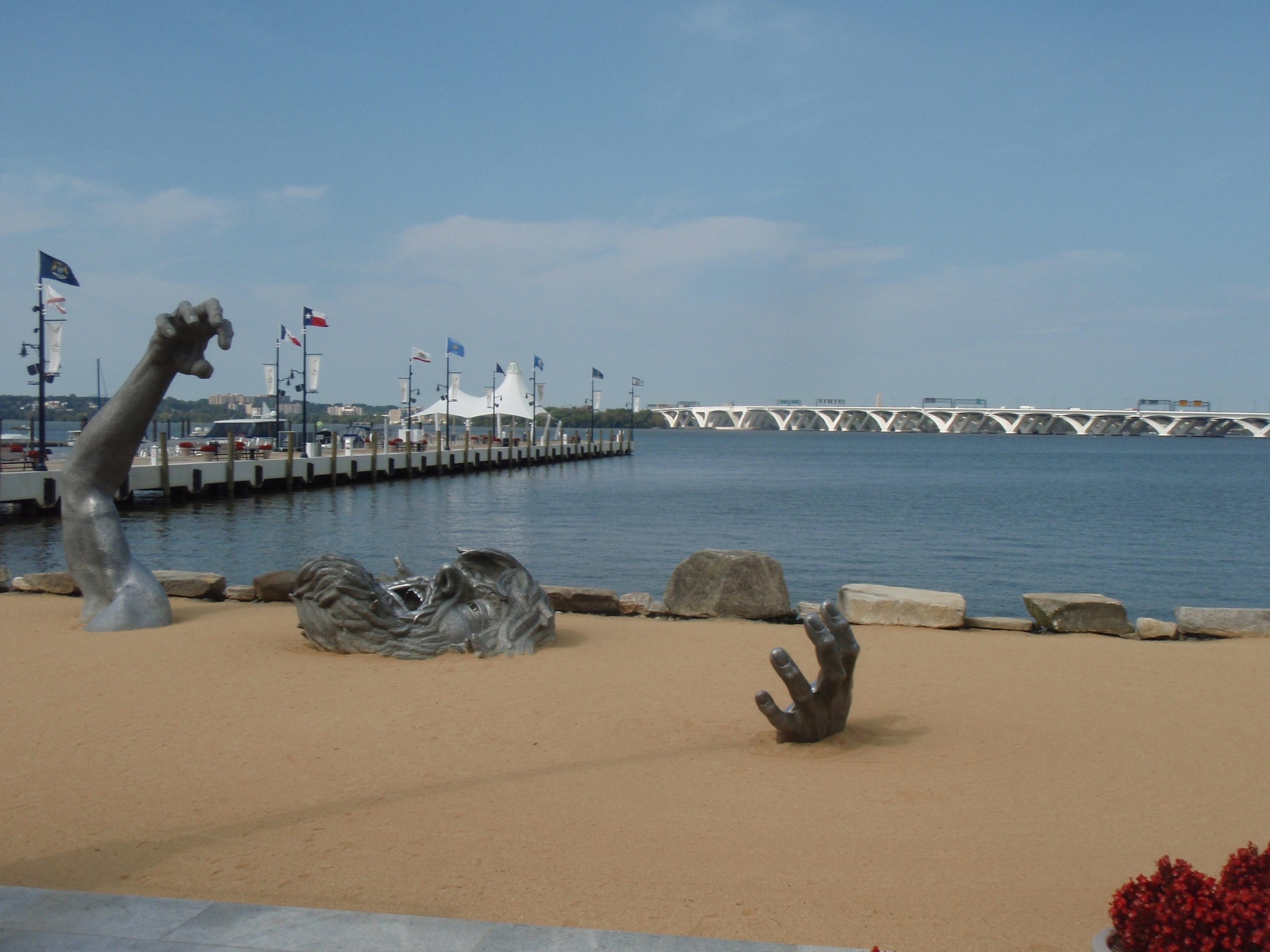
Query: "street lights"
487 395 503 437
435 384 458 450
525 392 543 446
18 342 48 472
626 402 635 441
290 368 309 457
584 398 600 443
407 388 421 428
275 376 291 451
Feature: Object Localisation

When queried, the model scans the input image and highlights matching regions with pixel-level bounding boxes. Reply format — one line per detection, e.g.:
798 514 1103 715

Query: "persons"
59 297 862 742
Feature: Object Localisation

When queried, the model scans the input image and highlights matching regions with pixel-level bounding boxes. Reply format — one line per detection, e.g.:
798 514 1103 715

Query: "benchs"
0 447 35 471
211 442 236 462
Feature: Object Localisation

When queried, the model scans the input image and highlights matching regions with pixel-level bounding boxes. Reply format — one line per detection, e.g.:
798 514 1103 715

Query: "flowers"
470 434 520 441
235 441 245 450
7 444 23 452
422 440 428 444
259 445 271 450
410 441 414 445
28 450 47 458
365 438 370 442
388 438 403 445
200 445 216 452
179 442 192 447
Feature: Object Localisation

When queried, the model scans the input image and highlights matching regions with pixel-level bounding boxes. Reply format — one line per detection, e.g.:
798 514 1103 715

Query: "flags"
38 250 80 288
632 377 643 386
534 355 543 371
303 307 329 327
447 337 464 357
280 324 302 346
592 368 604 379
496 363 506 376
46 286 67 314
412 347 431 363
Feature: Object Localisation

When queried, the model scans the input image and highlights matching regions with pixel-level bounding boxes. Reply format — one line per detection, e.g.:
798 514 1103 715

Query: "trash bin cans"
305 442 321 457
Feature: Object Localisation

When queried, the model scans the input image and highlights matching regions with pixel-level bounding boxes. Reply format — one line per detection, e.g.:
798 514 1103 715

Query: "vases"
423 444 427 451
388 441 403 453
180 447 191 457
201 452 214 461
263 450 272 459
467 439 524 446
34 458 46 469
236 450 242 460
410 445 413 451
364 443 372 450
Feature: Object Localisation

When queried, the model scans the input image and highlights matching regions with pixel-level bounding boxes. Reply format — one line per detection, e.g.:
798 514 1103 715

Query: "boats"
0 425 38 460
201 418 291 448
342 425 369 448
